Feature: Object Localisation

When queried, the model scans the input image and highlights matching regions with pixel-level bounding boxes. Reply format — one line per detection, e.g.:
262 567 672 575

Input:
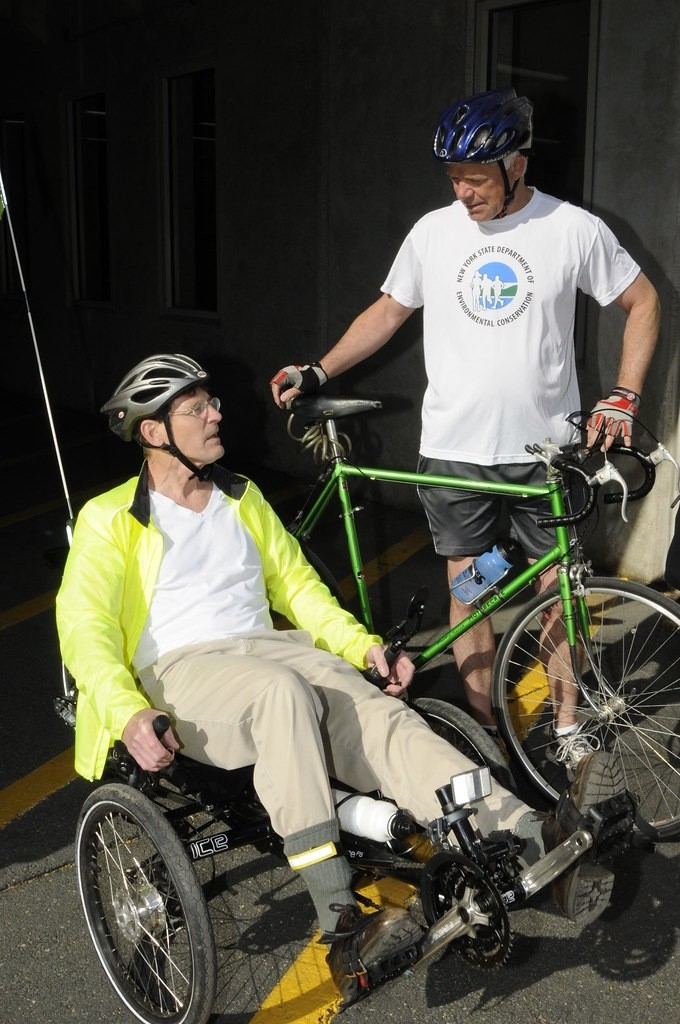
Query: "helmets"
100 354 211 441
432 87 534 162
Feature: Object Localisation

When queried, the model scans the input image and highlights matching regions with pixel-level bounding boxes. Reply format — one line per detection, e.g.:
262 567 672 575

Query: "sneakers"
315 901 424 1001
532 751 627 927
545 722 602 782
459 726 509 766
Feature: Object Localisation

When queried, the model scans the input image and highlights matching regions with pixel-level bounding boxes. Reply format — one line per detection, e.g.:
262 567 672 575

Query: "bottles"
331 788 416 843
451 543 520 604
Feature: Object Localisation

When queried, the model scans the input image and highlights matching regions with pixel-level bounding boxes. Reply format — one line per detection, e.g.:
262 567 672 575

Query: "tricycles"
52 510 631 1024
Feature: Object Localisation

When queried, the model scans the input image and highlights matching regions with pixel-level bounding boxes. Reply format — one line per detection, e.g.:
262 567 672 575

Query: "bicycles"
269 387 680 842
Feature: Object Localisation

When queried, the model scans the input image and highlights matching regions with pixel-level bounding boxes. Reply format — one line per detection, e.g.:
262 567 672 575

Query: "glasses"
168 397 220 418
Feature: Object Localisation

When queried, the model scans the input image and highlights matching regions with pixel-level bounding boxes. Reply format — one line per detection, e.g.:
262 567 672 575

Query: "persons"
54 355 628 1004
269 87 661 784
